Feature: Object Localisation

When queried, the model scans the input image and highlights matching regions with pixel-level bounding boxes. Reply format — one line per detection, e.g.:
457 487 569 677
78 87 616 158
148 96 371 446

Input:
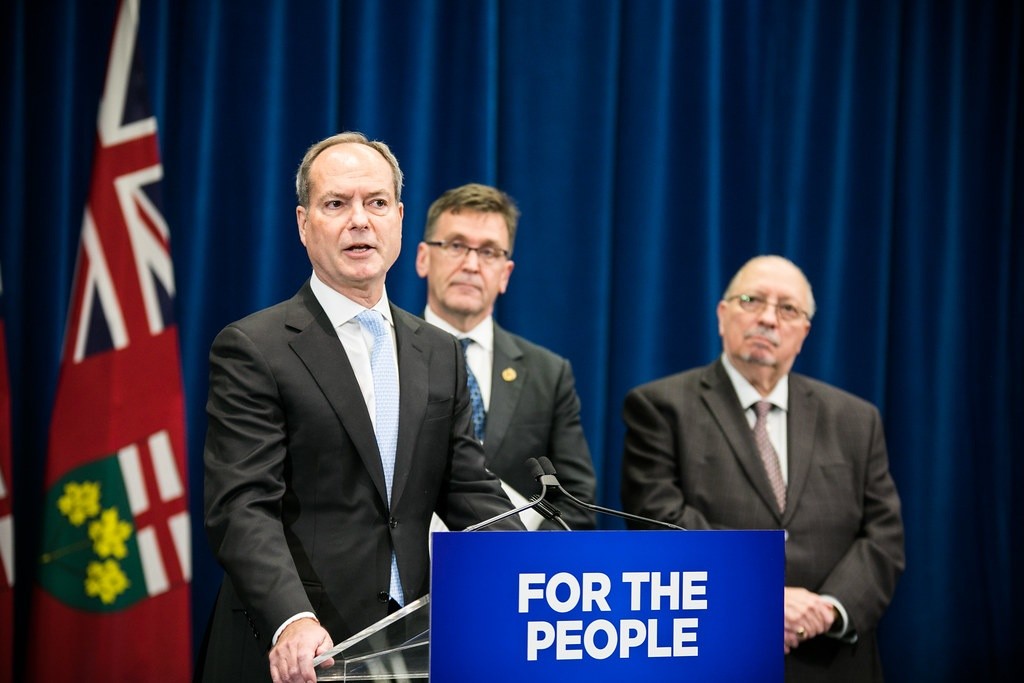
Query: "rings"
795 628 806 638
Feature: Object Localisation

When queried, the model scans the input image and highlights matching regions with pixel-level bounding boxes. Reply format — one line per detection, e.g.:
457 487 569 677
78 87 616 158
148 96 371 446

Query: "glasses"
728 294 810 322
427 240 508 263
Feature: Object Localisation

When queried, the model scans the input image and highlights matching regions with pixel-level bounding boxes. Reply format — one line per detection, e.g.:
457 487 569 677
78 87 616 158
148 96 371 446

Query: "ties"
355 309 404 608
459 337 487 445
750 401 786 517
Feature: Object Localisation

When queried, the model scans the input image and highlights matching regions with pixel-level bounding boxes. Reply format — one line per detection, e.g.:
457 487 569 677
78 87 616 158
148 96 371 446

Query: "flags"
1 286 26 682
32 1 199 683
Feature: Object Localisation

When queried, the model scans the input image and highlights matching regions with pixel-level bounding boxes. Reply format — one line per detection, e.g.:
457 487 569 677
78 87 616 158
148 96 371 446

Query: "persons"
410 185 598 593
624 254 905 682
203 135 534 683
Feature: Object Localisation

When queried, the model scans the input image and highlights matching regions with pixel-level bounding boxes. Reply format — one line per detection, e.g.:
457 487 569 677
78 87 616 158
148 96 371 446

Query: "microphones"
460 456 686 530
